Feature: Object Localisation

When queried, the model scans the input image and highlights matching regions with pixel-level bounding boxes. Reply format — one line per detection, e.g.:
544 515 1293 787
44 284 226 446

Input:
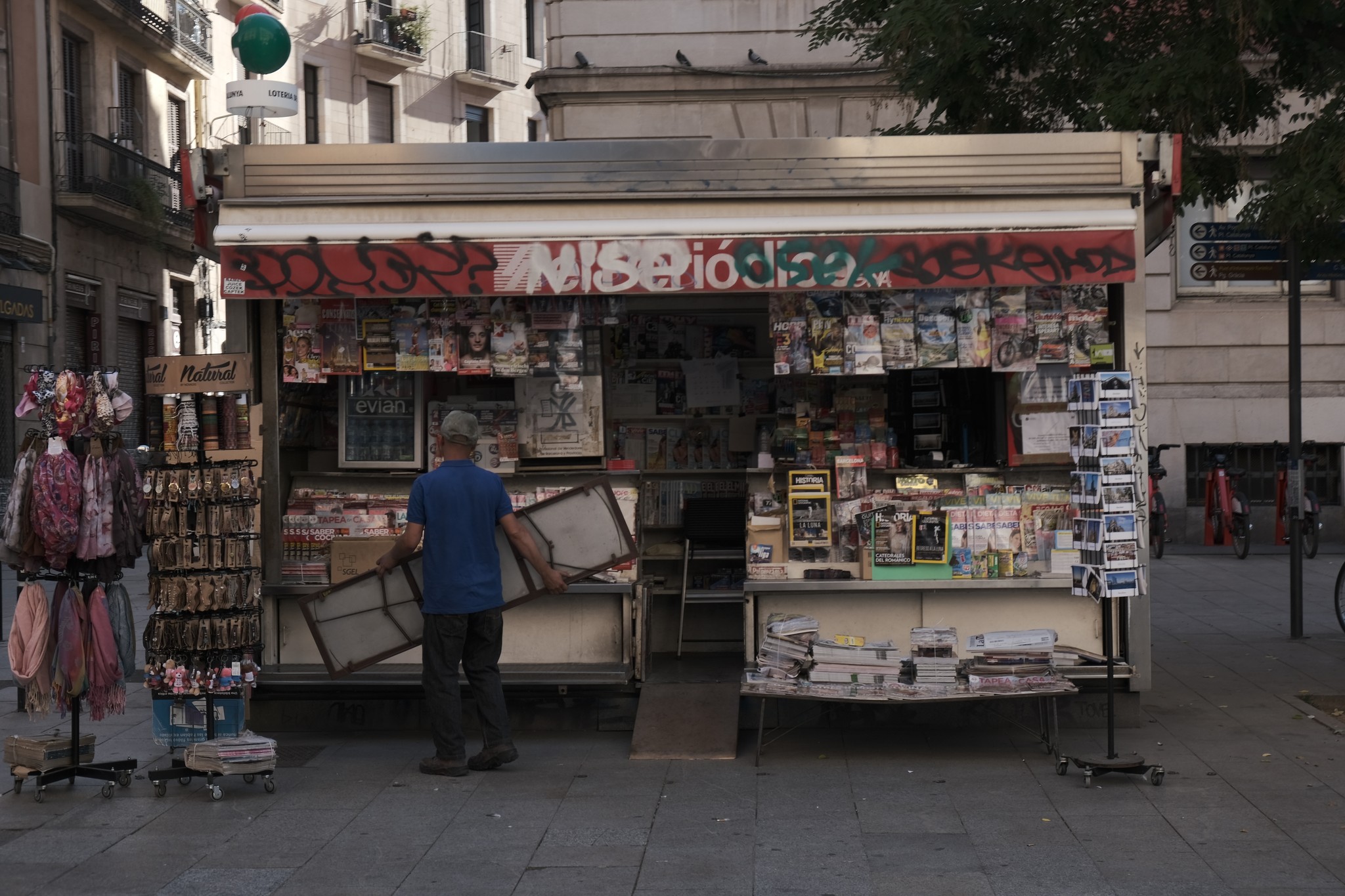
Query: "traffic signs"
1304 222 1345 241
1300 261 1345 281
1188 221 1293 241
1188 242 1291 261
1190 261 1289 282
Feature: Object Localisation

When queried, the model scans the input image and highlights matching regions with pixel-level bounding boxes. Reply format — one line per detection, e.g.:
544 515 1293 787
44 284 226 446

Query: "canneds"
886 446 899 469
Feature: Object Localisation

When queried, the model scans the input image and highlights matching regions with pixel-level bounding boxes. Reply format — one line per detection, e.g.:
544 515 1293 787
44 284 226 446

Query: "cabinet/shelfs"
268 292 1136 688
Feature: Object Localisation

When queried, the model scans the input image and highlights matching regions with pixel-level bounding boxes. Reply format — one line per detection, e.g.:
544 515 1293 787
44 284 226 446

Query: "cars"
1332 563 1345 635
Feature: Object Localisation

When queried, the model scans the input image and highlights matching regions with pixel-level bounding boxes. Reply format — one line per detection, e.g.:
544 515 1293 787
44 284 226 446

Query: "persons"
283 335 295 366
433 324 441 338
434 359 443 371
430 359 435 371
442 328 455 364
409 321 426 357
376 410 572 779
459 323 491 369
296 336 320 372
287 368 299 379
315 324 320 332
960 530 967 547
1008 528 1021 553
985 529 997 554
888 519 910 562
815 321 843 373
300 368 307 382
283 366 290 377
970 309 991 367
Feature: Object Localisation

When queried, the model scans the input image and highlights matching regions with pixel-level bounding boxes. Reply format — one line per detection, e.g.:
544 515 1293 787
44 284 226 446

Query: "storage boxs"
149 689 248 753
328 533 402 586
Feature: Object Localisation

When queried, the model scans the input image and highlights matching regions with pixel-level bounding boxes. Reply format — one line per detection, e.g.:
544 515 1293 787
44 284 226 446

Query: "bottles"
345 419 413 462
758 425 771 454
886 427 897 447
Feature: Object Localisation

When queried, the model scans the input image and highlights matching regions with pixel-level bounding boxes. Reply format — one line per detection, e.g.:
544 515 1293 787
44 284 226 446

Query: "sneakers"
419 754 468 776
467 740 519 772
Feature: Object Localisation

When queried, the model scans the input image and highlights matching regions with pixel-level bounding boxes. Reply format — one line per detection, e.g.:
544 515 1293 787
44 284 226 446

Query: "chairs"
676 494 747 660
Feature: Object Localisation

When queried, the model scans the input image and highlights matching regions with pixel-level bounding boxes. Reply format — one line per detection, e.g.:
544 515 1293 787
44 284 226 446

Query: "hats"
440 409 479 446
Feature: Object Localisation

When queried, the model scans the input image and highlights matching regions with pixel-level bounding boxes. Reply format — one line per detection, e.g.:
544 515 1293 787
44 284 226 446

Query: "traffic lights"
1302 242 1345 260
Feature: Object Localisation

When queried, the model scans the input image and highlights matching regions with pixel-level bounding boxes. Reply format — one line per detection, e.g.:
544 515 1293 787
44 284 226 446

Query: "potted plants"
388 6 430 56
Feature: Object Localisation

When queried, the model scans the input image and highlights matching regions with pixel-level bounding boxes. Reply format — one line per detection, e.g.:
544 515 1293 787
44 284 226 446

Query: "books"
282 295 611 384
752 284 1110 689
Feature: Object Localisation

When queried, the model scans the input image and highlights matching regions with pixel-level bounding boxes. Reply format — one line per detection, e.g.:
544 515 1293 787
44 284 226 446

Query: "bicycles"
1273 438 1322 559
1201 438 1253 559
1145 443 1183 558
